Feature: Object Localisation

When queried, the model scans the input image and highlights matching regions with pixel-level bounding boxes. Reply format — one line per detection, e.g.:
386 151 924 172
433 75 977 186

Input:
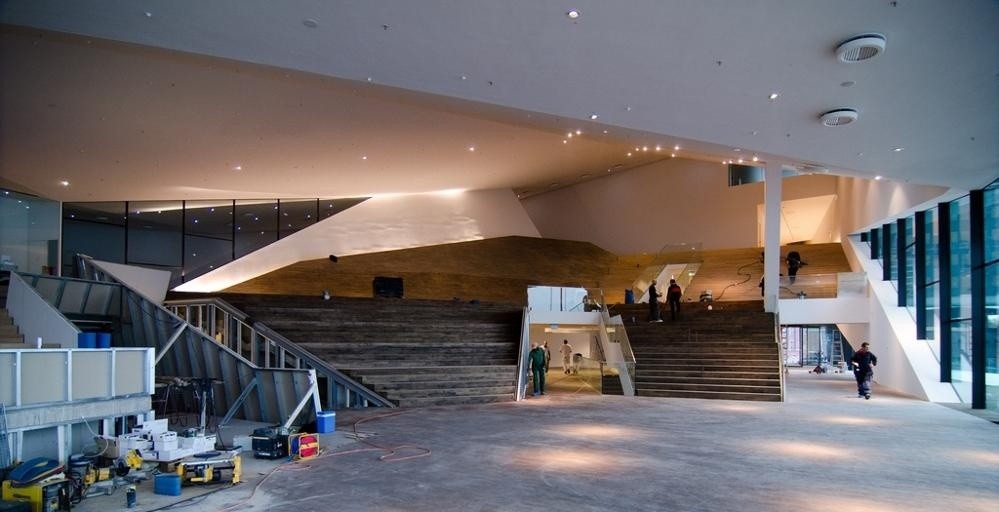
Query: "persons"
527 342 548 396
667 279 682 318
851 341 877 399
539 340 551 372
649 278 663 323
786 250 801 284
758 271 764 300
560 339 572 373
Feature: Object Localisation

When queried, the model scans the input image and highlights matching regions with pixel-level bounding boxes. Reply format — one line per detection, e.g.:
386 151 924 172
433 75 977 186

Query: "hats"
532 343 538 349
